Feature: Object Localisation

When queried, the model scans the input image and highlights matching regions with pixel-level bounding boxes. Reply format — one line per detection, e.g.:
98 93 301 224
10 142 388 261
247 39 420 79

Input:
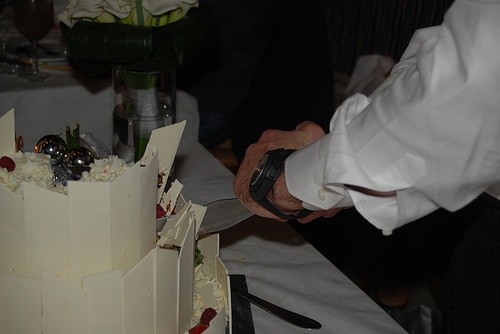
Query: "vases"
106 64 176 162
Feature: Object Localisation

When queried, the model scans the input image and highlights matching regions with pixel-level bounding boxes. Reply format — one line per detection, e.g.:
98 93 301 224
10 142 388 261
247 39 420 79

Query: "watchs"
232 0 500 237
247 147 313 221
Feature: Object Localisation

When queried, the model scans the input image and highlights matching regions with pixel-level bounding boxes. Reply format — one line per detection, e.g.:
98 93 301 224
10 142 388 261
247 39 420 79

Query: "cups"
110 62 180 182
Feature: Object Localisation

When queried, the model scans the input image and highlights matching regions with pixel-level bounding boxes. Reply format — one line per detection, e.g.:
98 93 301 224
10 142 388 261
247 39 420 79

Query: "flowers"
57 0 199 163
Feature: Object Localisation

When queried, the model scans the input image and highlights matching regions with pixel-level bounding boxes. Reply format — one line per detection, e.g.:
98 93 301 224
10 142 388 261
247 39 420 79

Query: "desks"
163 137 411 334
0 2 201 154
